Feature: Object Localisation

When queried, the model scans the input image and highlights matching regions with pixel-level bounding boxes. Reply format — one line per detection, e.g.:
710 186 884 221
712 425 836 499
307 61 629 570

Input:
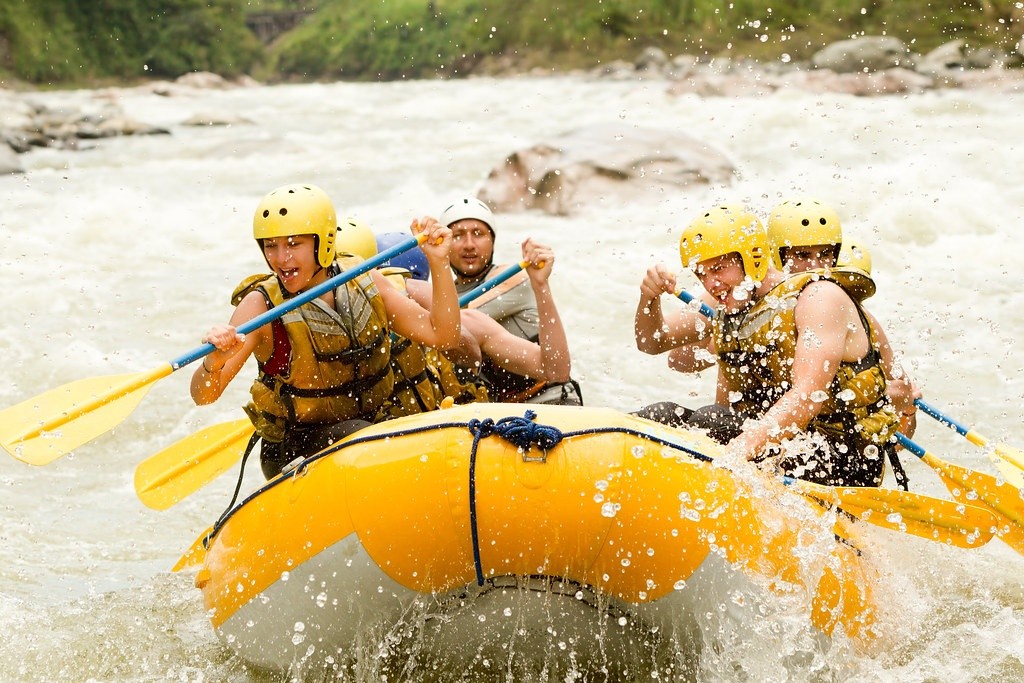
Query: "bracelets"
902 411 915 417
202 356 225 374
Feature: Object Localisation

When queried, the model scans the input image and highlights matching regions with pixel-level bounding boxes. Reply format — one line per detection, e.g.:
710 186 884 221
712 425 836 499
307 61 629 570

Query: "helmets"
334 219 378 271
767 200 843 274
680 206 770 284
375 233 430 281
836 242 872 276
253 183 336 274
439 196 497 239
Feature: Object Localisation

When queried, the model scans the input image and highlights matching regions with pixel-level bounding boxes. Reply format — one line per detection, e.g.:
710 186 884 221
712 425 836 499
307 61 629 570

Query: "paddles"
0 231 444 469
130 242 546 514
772 474 999 550
912 398 1023 492
672 286 1023 557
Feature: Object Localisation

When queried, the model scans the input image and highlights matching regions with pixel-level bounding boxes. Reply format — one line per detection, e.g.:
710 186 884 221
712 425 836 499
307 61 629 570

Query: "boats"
195 398 884 682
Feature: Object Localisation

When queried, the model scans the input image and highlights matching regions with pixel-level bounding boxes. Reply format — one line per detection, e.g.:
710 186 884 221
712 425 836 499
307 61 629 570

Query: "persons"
189 189 572 486
634 204 925 502
